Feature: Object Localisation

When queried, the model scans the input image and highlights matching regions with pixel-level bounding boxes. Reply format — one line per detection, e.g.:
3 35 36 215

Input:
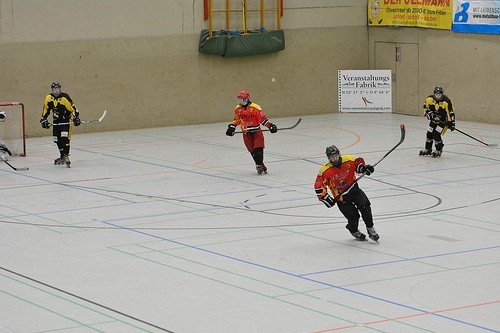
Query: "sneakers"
54 157 65 165
433 151 442 157
351 229 368 241
419 149 432 156
65 160 71 168
256 164 267 175
366 227 379 241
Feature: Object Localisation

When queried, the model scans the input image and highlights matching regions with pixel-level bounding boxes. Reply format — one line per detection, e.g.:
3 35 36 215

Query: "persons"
314 145 380 242
40 82 81 168
226 91 278 176
418 86 455 158
0 111 13 161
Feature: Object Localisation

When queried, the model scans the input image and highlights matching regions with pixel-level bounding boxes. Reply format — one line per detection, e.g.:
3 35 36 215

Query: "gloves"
226 124 236 136
40 118 50 129
451 120 455 131
73 118 81 126
431 112 441 121
363 164 374 175
269 123 277 133
321 193 335 208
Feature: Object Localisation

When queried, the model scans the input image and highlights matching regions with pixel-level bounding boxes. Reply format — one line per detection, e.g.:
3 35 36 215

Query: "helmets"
237 89 250 105
326 145 342 166
434 86 444 102
51 82 62 97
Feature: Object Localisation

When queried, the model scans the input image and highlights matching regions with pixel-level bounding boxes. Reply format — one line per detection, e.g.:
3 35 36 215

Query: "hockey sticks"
334 124 406 200
0 157 30 172
439 120 497 147
235 117 302 133
48 110 107 126
